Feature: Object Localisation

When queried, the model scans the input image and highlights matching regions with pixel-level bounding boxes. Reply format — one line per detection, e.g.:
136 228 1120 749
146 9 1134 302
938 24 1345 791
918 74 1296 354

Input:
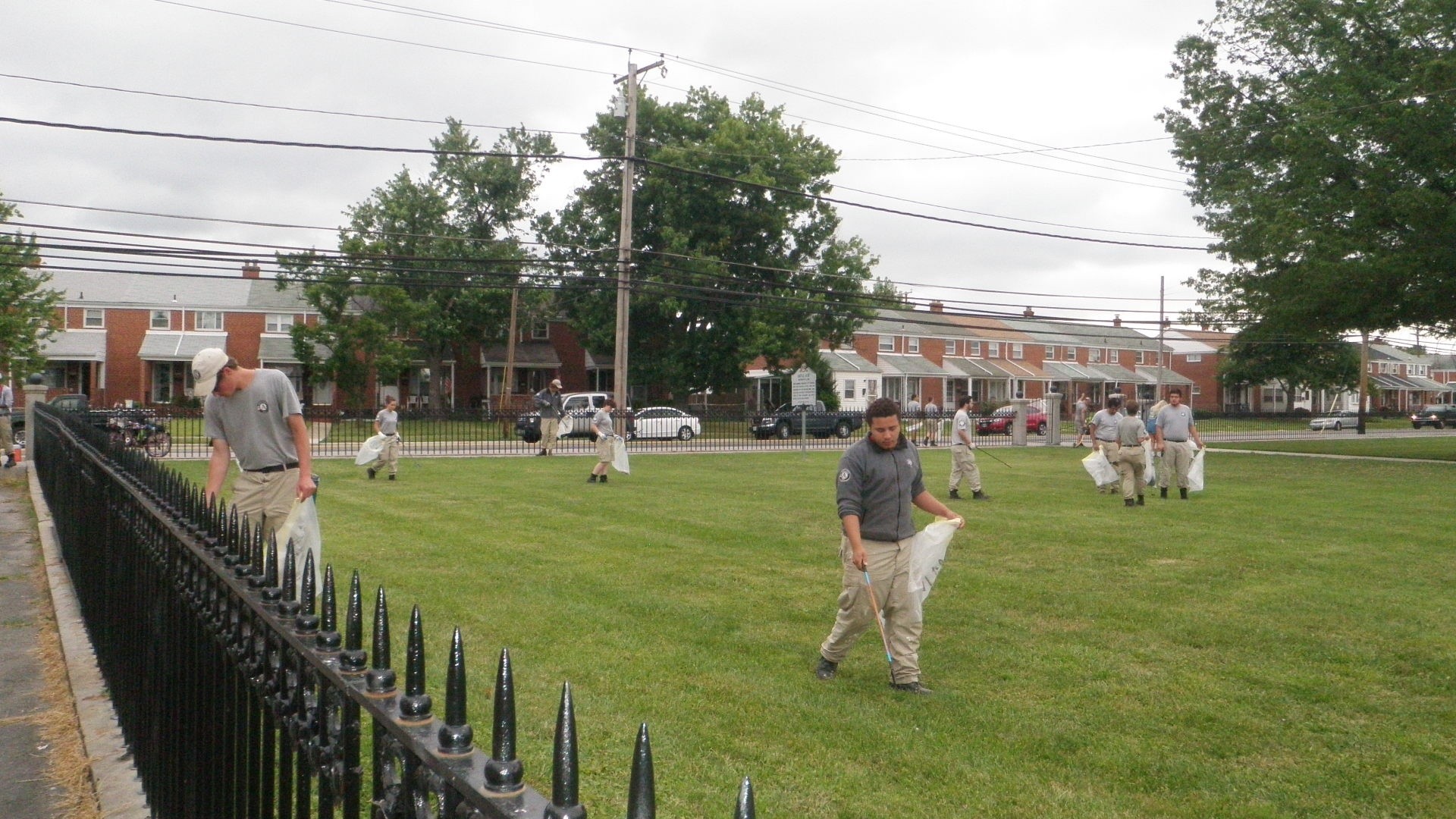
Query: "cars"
1410 404 1456 429
1310 410 1358 431
623 406 701 441
974 405 1047 438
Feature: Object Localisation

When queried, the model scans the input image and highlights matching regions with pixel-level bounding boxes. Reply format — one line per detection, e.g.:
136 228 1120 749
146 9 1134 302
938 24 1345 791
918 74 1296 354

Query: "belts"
1164 438 1186 442
387 434 393 436
247 462 299 472
1096 438 1115 442
1122 445 1141 446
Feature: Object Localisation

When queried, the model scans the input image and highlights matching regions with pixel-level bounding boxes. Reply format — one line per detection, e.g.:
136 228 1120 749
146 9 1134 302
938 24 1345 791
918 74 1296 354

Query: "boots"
1137 495 1144 505
972 490 988 499
599 475 608 483
950 489 961 499
537 449 546 456
912 437 938 446
5 454 16 467
1160 487 1166 498
1180 488 1187 499
587 473 597 483
1124 498 1134 505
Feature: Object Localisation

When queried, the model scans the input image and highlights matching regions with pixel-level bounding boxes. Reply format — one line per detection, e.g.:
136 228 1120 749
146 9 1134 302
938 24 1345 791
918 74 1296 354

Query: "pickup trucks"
750 401 863 439
12 394 145 447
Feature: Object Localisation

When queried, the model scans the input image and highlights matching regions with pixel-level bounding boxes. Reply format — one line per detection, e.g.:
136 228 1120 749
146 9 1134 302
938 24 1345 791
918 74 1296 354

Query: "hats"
191 348 229 396
552 380 563 388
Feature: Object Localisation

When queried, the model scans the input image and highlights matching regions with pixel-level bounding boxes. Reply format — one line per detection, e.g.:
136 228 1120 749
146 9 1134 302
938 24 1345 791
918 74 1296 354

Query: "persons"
532 379 562 456
1090 397 1125 494
192 347 317 569
907 394 922 446
817 397 965 695
924 397 945 446
586 400 624 484
1155 388 1206 501
1073 387 1169 458
367 395 401 481
949 395 991 500
0 372 16 468
1115 399 1150 507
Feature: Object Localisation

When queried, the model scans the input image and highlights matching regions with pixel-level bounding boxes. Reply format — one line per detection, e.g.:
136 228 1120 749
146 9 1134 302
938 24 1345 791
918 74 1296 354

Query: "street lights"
440 360 458 414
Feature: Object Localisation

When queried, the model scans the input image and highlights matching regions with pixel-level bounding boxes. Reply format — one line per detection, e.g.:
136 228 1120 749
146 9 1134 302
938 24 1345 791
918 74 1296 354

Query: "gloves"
598 432 605 439
968 442 976 449
396 432 400 441
378 432 386 440
543 400 550 406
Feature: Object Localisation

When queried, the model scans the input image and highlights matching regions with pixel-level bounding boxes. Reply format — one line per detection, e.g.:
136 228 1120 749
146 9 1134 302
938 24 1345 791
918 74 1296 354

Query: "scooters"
107 407 172 460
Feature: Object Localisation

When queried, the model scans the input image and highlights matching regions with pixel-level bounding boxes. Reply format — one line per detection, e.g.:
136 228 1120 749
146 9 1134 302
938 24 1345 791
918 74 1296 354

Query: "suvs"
513 391 631 443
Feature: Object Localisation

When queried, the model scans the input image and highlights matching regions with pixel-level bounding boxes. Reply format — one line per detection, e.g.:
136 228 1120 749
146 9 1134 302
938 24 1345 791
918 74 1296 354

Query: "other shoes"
389 475 394 480
368 468 374 479
892 682 932 694
818 656 838 679
